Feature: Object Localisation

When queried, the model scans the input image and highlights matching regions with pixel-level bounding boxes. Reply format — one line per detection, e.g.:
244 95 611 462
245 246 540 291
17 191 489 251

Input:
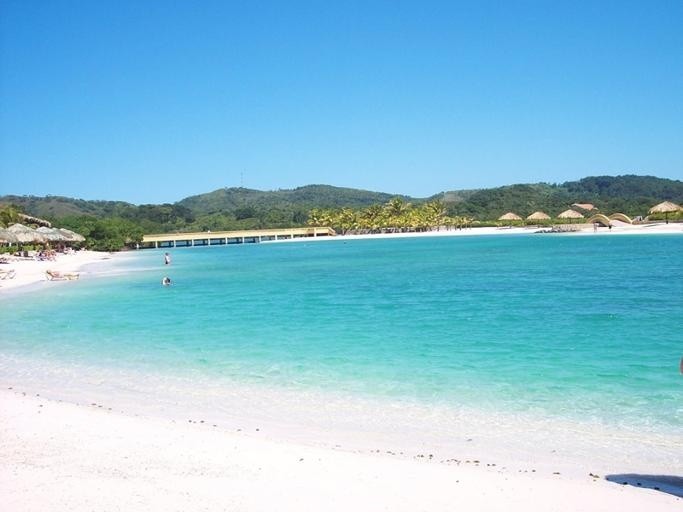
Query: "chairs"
36 256 56 262
0 269 16 281
44 270 80 282
0 257 11 264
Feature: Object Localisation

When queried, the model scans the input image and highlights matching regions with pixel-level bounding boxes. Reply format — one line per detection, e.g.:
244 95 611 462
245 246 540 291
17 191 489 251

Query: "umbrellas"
0 222 86 256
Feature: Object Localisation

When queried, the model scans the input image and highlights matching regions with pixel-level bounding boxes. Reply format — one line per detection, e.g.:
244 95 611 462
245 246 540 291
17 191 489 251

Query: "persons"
45 268 79 280
161 276 171 287
162 251 170 265
